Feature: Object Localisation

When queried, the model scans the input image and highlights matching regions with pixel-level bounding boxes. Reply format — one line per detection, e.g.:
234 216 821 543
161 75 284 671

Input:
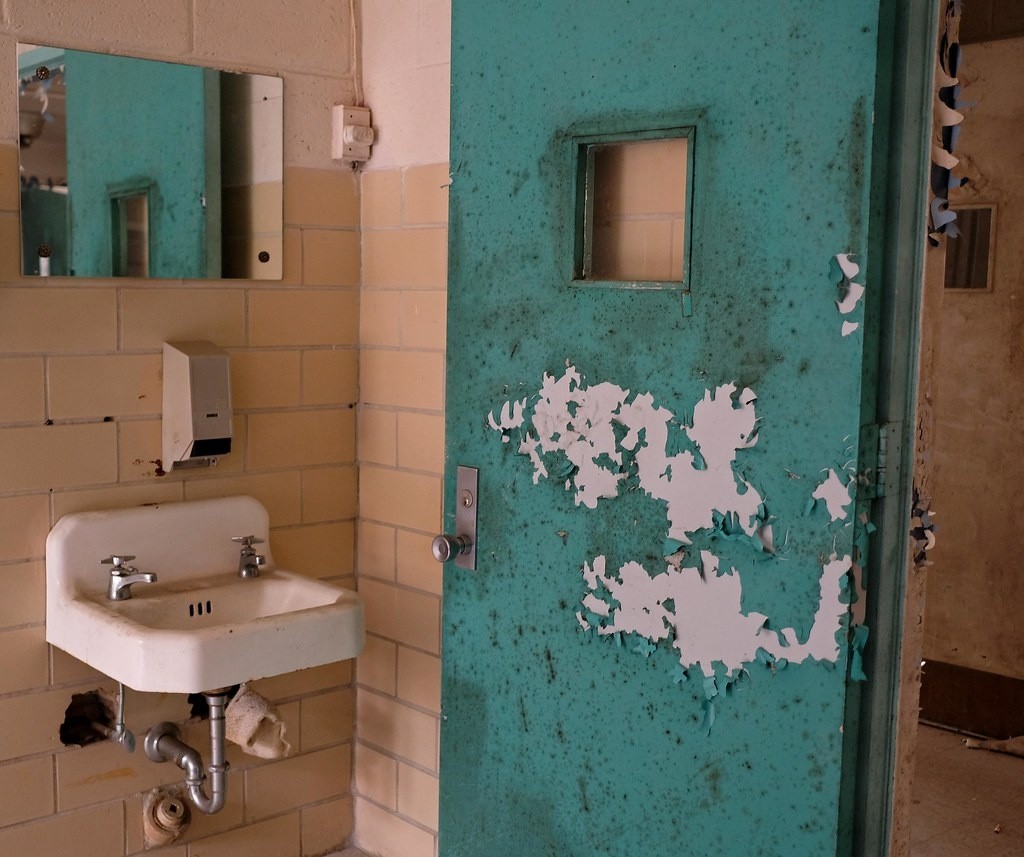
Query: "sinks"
42 494 370 695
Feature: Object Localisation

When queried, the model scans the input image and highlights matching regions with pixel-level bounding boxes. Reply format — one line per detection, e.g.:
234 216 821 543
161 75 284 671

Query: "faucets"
98 553 159 600
231 534 266 577
